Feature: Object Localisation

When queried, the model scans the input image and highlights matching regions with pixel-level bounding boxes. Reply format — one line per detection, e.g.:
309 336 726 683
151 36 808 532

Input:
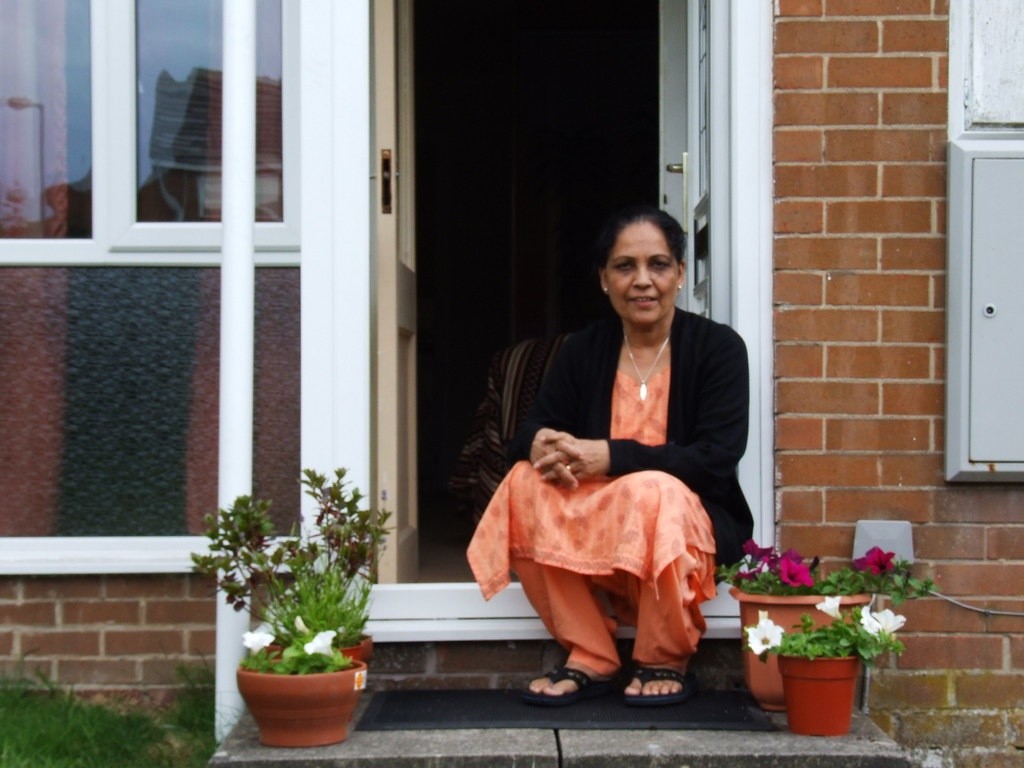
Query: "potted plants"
190 467 397 665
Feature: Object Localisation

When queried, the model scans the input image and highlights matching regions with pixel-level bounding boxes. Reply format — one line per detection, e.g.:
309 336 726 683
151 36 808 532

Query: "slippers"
521 665 625 706
622 666 698 706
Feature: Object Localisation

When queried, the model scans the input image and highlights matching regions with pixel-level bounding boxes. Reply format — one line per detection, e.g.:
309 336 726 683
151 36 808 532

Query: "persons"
466 207 754 704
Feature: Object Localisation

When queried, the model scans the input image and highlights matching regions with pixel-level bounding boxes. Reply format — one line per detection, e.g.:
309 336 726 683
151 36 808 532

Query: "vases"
235 660 367 747
728 586 874 712
777 655 861 736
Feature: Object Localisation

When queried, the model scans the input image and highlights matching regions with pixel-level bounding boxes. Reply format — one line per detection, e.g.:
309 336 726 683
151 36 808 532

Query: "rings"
566 465 570 470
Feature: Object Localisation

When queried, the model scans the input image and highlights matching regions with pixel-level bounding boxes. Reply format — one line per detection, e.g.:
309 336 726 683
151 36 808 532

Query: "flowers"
743 594 907 670
716 538 943 608
238 613 352 675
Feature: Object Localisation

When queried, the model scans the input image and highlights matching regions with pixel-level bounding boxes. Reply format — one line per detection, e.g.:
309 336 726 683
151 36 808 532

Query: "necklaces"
623 330 669 400
